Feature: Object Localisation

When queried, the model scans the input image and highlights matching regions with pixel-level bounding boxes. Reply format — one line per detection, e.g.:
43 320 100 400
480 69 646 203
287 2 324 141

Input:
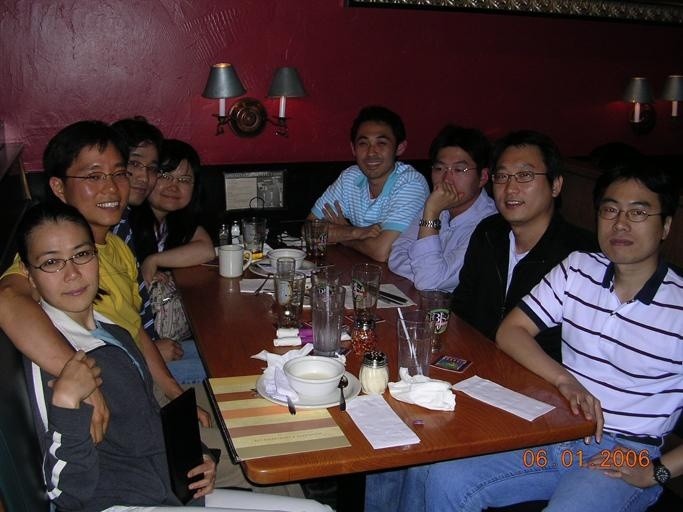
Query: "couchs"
26 159 435 241
0 335 49 512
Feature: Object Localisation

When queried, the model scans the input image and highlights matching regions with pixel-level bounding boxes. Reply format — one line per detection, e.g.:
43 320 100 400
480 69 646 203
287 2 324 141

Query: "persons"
386 125 499 293
364 131 603 512
423 159 683 512
1 116 335 511
306 106 429 263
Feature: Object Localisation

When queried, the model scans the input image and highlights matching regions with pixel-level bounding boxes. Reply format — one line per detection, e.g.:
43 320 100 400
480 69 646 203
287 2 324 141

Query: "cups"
242 217 267 258
351 261 382 332
217 245 252 279
276 257 295 286
396 309 436 382
303 219 328 257
272 271 306 322
309 268 343 291
416 286 453 354
310 284 345 357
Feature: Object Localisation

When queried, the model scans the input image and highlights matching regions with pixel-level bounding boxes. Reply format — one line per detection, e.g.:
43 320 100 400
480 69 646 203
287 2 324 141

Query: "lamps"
200 63 247 136
661 76 683 129
623 77 654 129
265 66 309 139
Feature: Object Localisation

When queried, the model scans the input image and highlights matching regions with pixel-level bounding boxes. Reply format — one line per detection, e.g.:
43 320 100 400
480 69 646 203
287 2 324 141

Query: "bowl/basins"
284 354 346 395
267 249 307 270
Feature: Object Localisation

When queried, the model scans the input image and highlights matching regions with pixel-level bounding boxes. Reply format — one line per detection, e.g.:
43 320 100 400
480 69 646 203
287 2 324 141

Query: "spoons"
252 272 273 296
338 374 349 411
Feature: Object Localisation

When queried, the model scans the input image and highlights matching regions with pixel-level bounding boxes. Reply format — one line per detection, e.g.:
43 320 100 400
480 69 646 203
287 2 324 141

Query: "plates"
256 366 361 409
248 259 317 278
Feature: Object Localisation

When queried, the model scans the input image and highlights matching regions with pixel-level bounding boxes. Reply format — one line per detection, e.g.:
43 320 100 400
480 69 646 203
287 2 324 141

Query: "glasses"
25 248 97 273
159 173 193 185
127 160 163 179
596 206 661 223
431 164 477 176
65 170 132 183
490 171 548 184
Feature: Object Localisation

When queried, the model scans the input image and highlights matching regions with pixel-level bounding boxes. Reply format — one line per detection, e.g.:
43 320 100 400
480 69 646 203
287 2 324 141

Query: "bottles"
350 316 378 358
217 223 229 245
230 221 241 245
358 351 390 397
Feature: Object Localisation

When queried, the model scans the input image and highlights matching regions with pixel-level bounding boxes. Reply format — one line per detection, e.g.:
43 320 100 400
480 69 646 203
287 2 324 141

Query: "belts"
604 432 661 447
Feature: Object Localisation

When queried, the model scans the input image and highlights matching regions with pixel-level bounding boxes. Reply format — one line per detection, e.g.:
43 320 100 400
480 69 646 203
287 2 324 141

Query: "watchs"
420 217 442 232
652 457 670 486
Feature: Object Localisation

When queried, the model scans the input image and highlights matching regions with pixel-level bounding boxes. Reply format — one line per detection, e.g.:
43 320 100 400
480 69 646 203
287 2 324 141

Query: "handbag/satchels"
148 270 191 342
